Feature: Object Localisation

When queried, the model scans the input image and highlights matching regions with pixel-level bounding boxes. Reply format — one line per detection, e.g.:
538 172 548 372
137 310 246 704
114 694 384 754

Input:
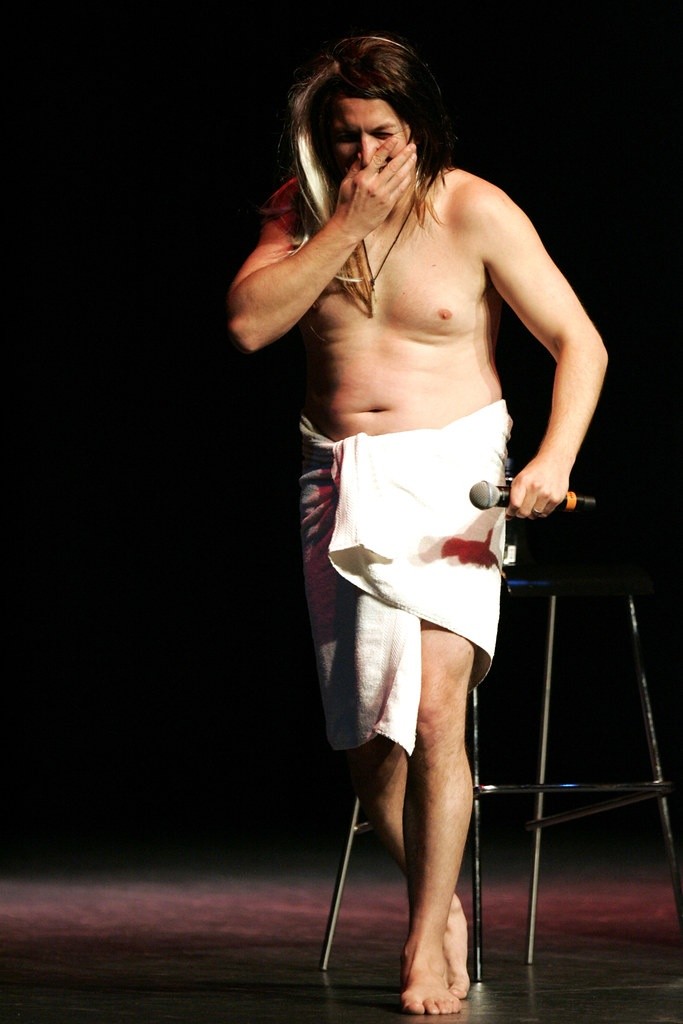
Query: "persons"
227 34 610 1015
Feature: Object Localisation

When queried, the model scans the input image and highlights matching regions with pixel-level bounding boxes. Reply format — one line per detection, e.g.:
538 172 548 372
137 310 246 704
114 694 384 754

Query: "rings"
532 508 542 516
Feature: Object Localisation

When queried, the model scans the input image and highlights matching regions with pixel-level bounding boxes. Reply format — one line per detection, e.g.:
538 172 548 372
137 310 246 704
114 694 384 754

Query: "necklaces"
361 200 415 316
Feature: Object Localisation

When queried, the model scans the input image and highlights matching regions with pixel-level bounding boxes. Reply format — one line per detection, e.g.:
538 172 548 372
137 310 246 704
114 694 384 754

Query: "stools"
316 563 683 985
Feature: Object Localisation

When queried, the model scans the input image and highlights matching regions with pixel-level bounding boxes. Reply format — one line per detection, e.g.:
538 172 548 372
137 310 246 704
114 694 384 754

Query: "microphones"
469 479 597 512
